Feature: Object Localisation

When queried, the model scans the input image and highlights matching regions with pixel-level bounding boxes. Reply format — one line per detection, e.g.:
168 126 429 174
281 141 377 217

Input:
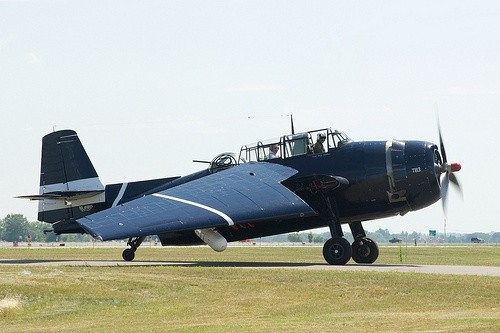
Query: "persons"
312 131 327 154
266 143 281 159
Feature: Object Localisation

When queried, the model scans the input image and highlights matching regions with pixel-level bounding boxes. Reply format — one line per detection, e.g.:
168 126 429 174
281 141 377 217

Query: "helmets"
316 132 325 140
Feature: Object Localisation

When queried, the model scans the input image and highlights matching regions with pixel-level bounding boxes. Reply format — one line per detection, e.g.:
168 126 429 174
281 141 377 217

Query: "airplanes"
14 115 462 266
